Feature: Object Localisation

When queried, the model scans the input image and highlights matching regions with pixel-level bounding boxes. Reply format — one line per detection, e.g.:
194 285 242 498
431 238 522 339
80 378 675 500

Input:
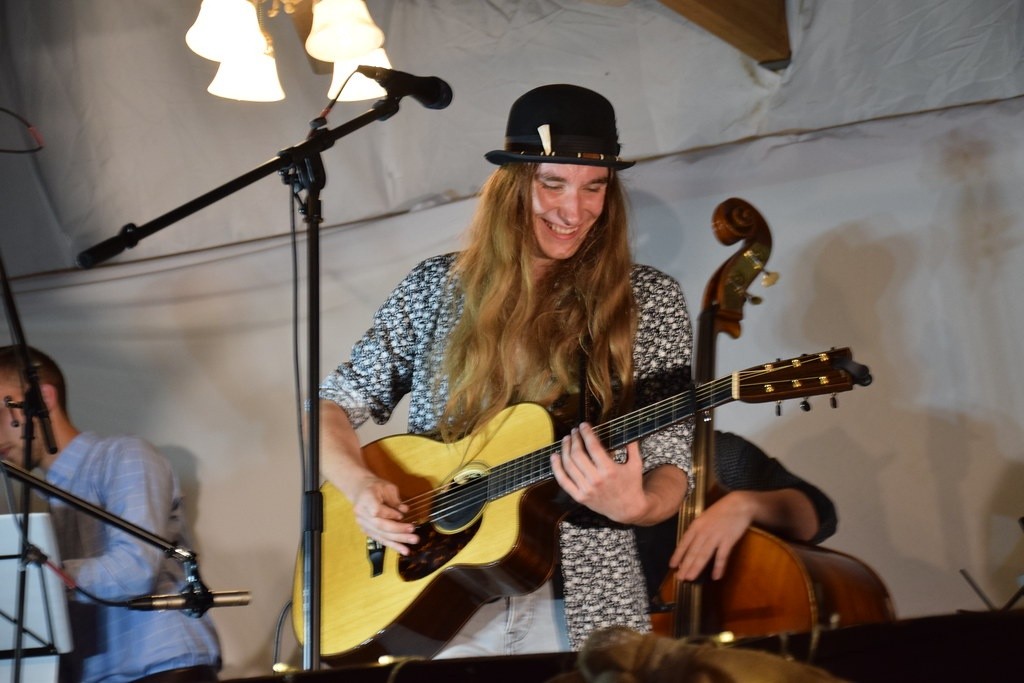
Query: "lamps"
206 5 286 103
326 48 392 101
185 0 268 62
305 0 384 62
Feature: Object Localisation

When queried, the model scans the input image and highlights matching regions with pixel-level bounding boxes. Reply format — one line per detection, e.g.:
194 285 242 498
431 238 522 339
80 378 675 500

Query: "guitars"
291 343 874 673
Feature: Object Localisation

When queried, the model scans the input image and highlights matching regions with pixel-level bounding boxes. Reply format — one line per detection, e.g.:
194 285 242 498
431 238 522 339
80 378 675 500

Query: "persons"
668 430 837 582
0 343 225 683
303 84 694 660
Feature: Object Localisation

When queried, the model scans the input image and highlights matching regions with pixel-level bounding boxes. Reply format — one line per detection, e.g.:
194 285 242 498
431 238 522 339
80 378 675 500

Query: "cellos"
644 193 899 645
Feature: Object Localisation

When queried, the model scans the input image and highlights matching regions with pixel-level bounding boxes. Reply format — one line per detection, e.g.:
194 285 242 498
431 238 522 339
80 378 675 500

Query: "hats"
484 84 635 173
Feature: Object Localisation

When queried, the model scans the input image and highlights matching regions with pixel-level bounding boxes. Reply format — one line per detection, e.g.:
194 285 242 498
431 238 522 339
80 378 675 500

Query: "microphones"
126 590 252 611
357 64 453 110
